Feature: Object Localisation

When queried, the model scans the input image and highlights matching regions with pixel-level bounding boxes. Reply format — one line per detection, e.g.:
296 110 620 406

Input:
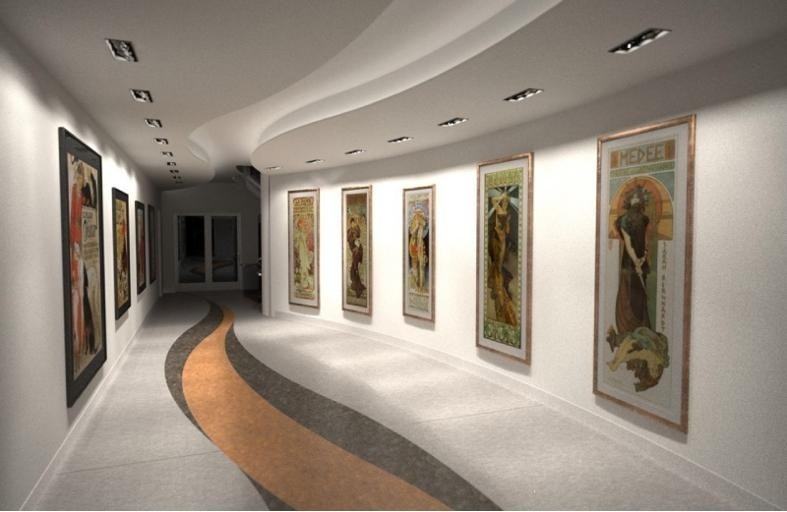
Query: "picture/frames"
285 181 439 333
591 110 699 438
472 149 536 375
54 124 159 411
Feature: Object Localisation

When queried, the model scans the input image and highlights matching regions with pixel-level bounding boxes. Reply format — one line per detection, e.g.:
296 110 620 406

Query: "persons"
615 190 649 332
489 192 511 303
